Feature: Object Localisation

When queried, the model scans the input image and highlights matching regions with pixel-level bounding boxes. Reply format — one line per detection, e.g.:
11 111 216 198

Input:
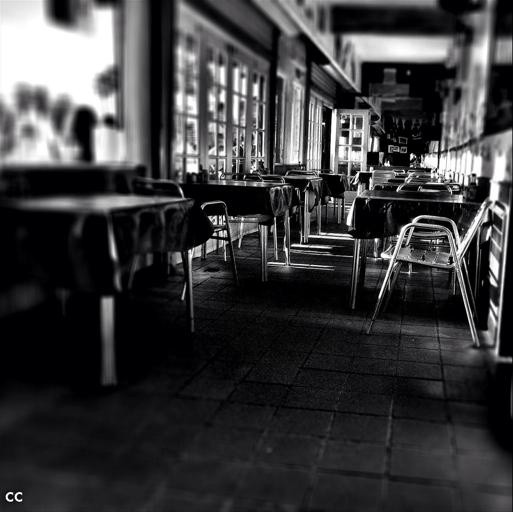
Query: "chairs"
110 163 494 348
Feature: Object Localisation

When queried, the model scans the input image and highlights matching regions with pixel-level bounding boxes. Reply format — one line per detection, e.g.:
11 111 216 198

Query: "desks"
1 191 196 387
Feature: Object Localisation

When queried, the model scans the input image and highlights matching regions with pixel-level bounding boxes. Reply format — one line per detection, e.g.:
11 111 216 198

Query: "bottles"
469 183 477 200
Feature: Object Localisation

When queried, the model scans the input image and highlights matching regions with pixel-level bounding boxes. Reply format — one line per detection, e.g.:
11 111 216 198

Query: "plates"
399 189 441 195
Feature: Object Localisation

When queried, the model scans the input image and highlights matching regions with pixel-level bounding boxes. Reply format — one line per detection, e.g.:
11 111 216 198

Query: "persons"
208 133 223 170
185 122 198 173
232 134 256 173
